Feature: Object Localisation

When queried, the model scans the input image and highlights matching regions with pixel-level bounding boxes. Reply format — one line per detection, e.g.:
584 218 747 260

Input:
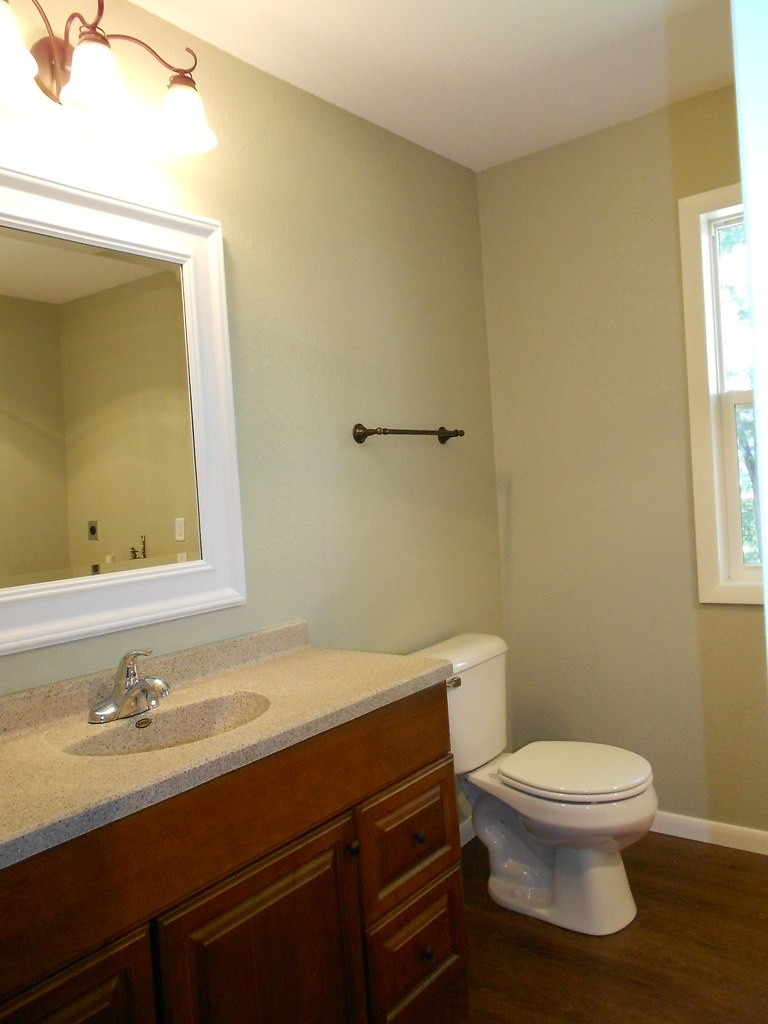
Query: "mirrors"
0 166 248 658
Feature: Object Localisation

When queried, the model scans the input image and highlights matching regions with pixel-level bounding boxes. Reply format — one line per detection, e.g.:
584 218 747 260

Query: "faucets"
87 649 171 720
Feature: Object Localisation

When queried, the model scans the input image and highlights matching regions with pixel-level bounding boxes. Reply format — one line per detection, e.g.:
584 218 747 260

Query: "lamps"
0 0 218 157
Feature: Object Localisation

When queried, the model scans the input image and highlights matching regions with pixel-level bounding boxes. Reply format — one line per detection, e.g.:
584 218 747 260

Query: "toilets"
403 633 659 935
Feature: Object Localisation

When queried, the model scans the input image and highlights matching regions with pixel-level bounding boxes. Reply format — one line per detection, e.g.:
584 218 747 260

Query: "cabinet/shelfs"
0 679 472 1024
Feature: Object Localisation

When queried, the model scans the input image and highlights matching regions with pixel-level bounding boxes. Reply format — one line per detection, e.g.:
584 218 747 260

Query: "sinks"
55 691 272 756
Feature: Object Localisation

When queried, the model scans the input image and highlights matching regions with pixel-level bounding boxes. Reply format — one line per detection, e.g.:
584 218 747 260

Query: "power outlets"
175 518 185 541
